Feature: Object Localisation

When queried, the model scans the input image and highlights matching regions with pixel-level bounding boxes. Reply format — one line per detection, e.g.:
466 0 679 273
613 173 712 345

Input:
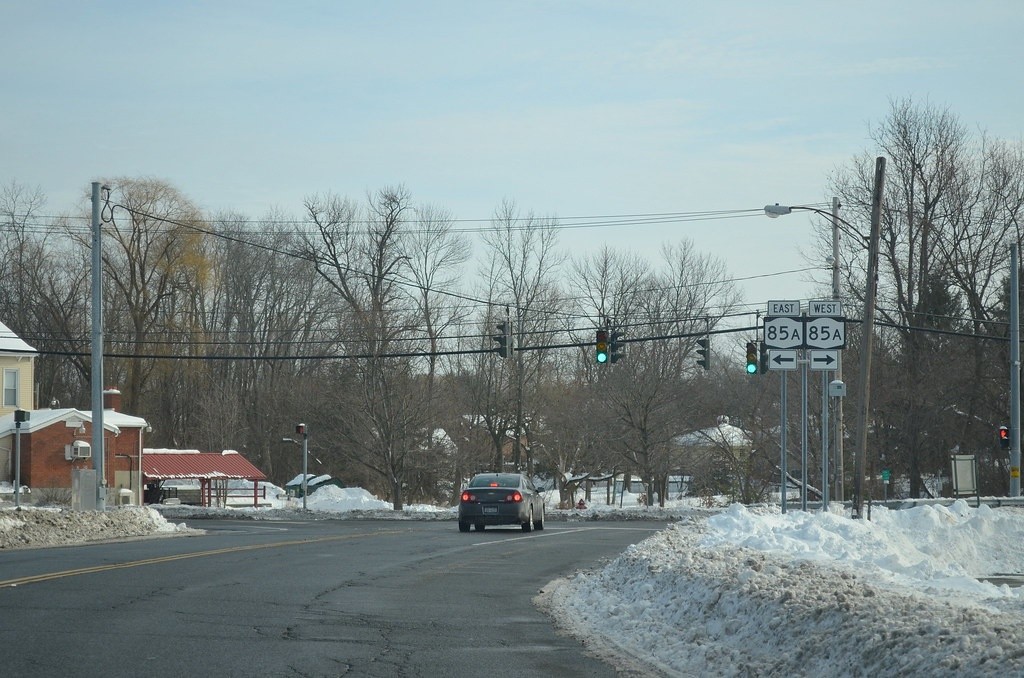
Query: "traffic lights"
746 342 758 376
597 329 608 365
610 330 625 365
696 338 710 371
492 322 513 358
296 424 306 434
999 427 1008 448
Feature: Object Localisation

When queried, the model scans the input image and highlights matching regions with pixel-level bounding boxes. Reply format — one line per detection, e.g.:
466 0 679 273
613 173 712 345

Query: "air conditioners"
70 446 91 458
115 493 135 508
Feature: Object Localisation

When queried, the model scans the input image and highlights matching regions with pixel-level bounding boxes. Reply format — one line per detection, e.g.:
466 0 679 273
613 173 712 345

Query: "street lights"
763 203 883 521
101 389 121 489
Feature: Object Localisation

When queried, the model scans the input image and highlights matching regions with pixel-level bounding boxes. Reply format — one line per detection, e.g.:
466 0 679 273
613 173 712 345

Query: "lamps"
73 423 86 436
142 421 152 433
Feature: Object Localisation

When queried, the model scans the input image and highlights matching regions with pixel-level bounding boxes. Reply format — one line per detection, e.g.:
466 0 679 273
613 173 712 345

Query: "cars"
458 472 547 533
